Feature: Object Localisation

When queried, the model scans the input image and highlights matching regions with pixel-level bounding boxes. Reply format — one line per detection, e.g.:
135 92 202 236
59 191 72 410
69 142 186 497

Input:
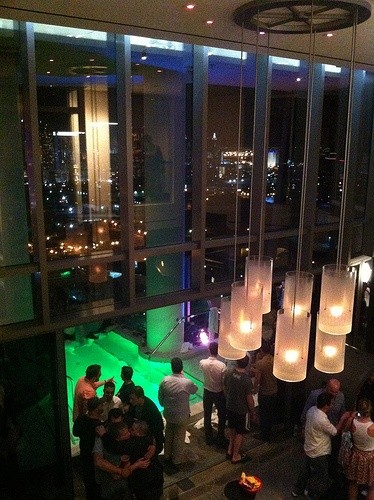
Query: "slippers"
233 452 248 463
227 453 231 456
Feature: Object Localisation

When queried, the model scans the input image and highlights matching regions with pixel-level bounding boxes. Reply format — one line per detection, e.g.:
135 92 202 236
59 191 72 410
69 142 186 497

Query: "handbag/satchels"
339 417 354 466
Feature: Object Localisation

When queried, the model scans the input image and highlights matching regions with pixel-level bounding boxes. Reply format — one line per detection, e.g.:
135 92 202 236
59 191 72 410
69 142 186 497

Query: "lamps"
216 0 371 382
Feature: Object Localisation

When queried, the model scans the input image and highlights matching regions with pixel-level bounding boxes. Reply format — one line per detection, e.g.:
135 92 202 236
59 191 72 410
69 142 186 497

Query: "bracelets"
105 380 106 384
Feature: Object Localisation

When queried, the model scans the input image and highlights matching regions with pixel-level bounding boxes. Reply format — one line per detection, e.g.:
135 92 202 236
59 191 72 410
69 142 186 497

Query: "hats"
88 396 100 411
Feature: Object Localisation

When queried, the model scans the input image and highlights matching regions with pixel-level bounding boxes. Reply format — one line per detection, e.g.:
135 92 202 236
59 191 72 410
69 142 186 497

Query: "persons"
254 343 278 441
199 342 229 446
338 371 374 500
300 379 345 481
224 354 254 464
158 357 198 469
73 365 164 500
292 392 351 500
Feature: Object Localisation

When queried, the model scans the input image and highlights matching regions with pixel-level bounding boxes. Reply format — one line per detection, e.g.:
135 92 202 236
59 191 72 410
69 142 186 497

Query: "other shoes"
292 490 309 497
